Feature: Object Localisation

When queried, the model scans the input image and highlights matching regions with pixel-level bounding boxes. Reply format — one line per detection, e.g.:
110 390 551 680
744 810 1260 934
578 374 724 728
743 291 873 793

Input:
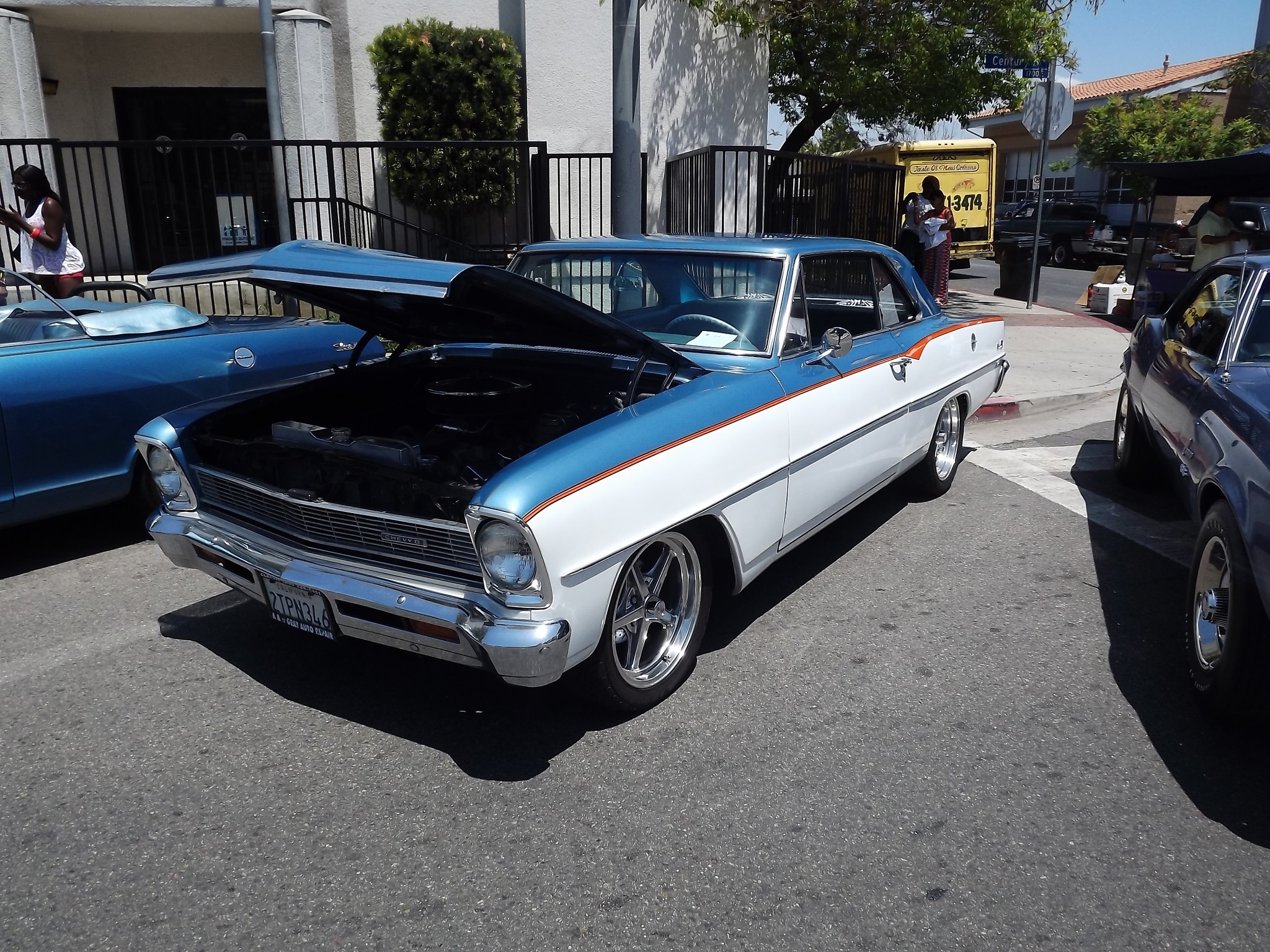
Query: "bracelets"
30 227 40 239
915 206 918 208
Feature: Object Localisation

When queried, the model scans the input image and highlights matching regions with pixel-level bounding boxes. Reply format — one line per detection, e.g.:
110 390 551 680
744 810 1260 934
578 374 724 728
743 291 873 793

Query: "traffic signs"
985 53 1050 79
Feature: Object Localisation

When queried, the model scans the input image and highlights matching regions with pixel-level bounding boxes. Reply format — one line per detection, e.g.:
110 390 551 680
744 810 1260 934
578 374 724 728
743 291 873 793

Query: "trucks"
801 138 995 272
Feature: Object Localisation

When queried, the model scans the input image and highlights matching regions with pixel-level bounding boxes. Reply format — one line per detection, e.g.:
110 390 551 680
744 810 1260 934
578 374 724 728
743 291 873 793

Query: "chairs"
1142 267 1196 312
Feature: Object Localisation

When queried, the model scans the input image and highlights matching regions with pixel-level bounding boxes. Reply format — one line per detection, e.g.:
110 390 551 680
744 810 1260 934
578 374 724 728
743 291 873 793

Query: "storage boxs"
1090 284 1134 314
1132 290 1163 320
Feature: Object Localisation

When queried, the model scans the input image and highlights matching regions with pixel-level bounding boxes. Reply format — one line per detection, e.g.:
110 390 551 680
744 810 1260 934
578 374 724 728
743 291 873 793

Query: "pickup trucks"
993 202 1129 267
1088 200 1270 271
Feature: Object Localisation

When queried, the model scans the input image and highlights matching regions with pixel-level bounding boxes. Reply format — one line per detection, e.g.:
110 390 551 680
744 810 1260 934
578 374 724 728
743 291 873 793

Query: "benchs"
0 318 61 343
673 299 873 351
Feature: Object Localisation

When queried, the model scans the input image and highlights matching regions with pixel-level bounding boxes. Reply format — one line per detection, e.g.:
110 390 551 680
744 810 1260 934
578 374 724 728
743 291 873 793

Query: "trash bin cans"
994 235 1051 303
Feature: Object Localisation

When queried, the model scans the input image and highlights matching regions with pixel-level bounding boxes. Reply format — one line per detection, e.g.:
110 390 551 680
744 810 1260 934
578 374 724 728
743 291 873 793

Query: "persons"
898 176 954 307
0 164 85 298
1191 193 1253 280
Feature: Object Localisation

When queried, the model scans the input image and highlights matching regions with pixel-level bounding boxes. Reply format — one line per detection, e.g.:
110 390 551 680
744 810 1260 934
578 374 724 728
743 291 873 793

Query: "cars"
131 238 1008 712
1 282 392 536
1113 250 1270 700
994 198 1072 218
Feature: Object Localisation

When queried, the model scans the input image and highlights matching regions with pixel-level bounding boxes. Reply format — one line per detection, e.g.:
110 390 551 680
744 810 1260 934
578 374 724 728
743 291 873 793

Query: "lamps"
41 77 59 96
